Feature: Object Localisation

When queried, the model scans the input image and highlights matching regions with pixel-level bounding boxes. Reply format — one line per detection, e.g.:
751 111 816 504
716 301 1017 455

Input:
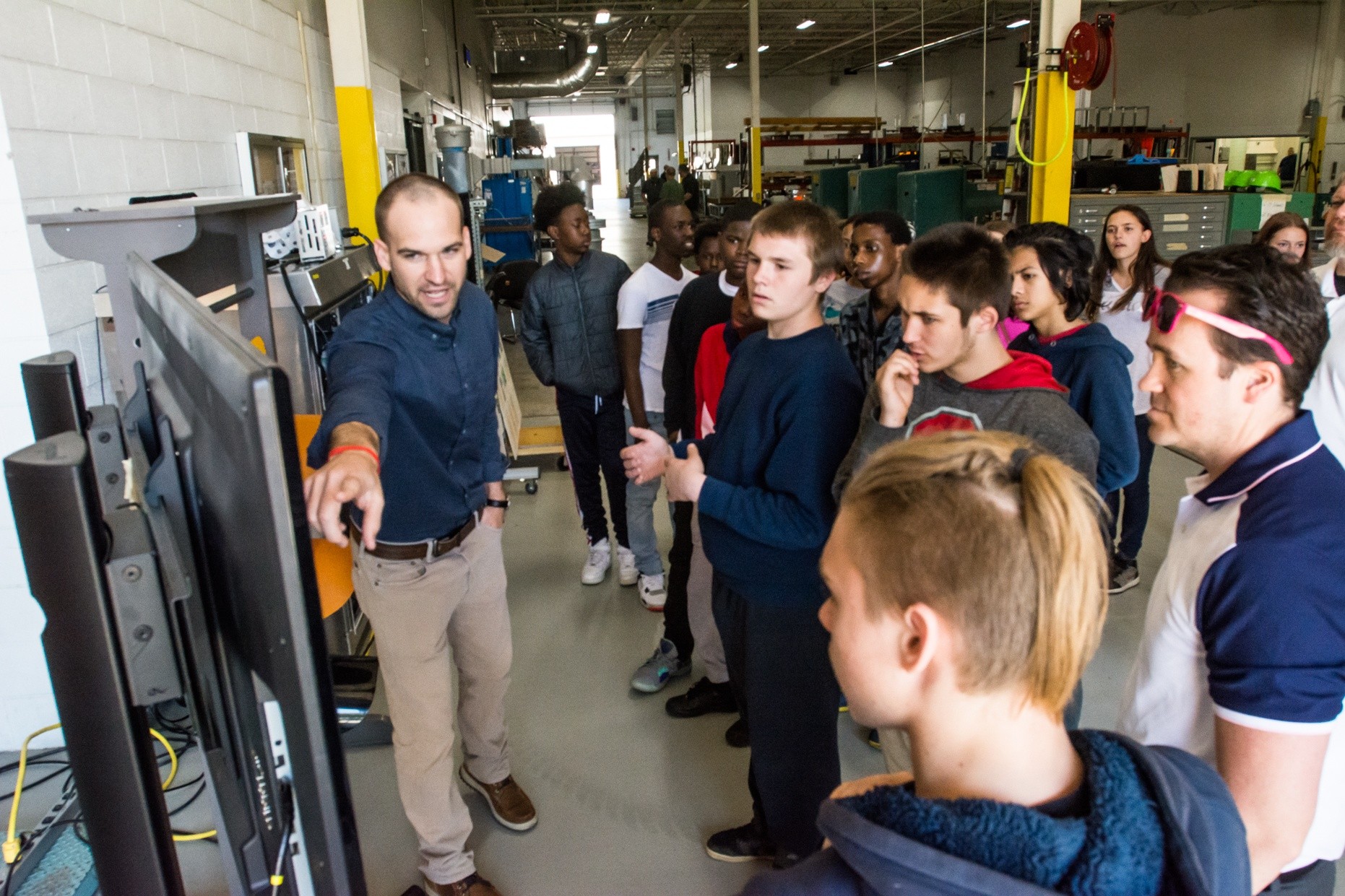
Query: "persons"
617 197 701 610
304 173 533 896
1084 206 1173 594
521 183 641 586
739 430 1255 896
622 206 866 868
1115 246 1345 896
640 165 700 247
1278 148 1296 180
628 197 1139 748
1254 176 1345 470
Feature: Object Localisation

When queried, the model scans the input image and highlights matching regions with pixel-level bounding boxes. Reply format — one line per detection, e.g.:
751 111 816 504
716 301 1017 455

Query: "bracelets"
326 446 380 476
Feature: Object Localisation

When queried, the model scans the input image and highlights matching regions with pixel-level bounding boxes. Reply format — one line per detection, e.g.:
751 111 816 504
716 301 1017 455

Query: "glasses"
1141 282 1297 366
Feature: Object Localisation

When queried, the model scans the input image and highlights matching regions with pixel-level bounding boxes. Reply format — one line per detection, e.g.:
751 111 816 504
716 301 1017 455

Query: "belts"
346 505 483 560
1263 859 1323 892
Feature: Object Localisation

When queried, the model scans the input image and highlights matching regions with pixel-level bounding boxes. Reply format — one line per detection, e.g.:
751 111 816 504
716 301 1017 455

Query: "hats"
650 169 657 173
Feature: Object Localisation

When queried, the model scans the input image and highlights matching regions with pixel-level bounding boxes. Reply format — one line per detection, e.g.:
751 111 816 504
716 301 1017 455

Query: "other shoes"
646 241 654 246
666 677 739 718
707 822 823 863
838 689 848 712
725 719 752 748
868 729 883 749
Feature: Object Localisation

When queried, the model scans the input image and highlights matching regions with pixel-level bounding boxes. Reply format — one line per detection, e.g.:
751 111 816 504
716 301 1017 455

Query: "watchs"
484 493 510 511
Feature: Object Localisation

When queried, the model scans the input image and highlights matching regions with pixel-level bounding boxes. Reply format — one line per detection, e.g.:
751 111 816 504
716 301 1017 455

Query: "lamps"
594 8 611 24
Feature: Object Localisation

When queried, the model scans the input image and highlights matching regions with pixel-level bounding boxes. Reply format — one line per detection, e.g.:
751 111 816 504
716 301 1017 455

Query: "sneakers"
631 638 692 694
459 761 539 831
1101 541 1140 593
637 570 668 612
423 871 501 896
581 538 612 585
616 543 639 585
